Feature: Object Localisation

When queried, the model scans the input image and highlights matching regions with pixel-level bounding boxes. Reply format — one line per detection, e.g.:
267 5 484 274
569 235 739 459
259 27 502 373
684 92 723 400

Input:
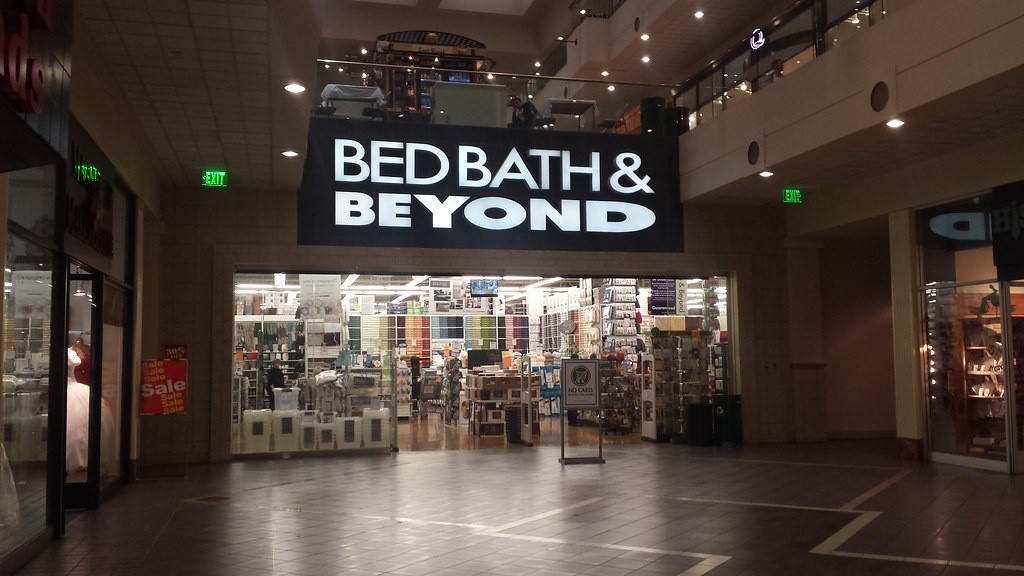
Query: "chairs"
311 95 336 117
362 108 386 120
535 118 555 130
597 113 628 134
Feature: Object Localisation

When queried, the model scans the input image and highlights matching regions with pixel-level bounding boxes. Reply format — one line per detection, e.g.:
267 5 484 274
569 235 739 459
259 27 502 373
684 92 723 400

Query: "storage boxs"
241 389 388 453
422 368 436 375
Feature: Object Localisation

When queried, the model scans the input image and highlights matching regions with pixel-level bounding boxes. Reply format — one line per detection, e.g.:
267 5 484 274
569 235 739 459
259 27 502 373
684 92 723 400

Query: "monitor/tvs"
470 279 499 298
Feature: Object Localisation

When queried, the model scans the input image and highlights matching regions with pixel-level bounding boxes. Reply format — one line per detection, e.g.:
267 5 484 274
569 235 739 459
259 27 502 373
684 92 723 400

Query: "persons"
68 338 90 385
67 349 115 475
768 59 785 82
512 98 537 129
265 359 287 411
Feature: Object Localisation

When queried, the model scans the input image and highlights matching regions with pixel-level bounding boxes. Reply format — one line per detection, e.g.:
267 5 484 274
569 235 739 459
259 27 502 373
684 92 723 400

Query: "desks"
549 98 599 132
320 84 386 120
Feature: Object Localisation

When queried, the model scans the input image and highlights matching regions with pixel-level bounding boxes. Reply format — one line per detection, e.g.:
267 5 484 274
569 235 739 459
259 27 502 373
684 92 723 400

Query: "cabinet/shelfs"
957 315 1024 462
373 50 485 114
233 278 731 443
